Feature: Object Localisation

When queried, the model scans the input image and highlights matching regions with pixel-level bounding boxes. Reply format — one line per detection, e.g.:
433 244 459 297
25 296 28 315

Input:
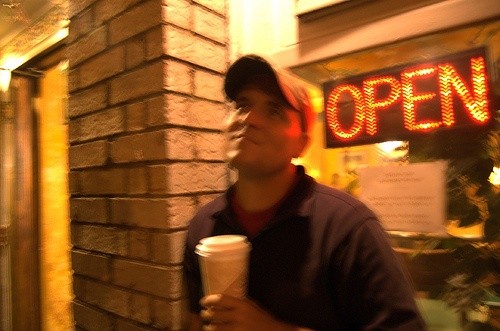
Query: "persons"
181 53 430 331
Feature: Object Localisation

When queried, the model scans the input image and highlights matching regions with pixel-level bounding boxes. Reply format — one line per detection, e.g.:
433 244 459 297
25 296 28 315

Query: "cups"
193 234 250 329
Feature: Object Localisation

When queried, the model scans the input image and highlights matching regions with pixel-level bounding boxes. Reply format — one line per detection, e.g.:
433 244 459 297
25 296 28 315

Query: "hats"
223 54 324 157
